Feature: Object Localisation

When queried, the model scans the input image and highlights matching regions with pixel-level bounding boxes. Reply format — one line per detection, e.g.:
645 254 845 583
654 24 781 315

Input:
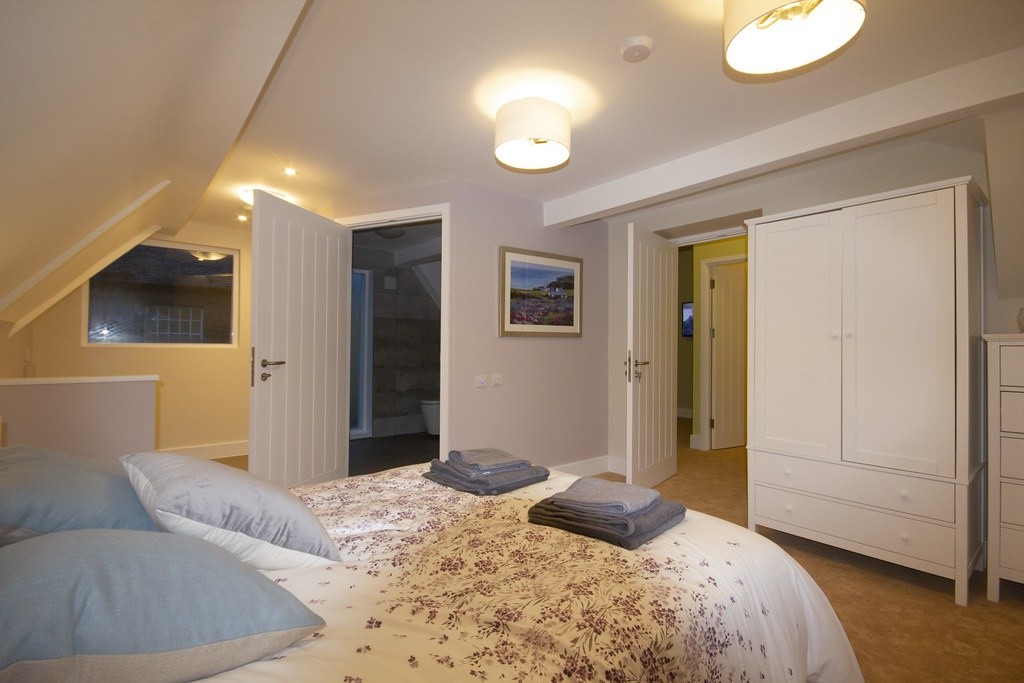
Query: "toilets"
418 400 440 435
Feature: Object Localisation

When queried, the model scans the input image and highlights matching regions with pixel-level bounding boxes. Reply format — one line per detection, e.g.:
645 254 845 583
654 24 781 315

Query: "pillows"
0 445 341 683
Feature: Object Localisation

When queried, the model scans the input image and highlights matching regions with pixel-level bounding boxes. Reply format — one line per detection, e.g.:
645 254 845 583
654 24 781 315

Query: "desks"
0 375 160 460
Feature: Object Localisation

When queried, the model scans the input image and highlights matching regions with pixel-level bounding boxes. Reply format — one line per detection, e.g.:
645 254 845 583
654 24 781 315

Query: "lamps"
724 0 866 74
494 97 571 169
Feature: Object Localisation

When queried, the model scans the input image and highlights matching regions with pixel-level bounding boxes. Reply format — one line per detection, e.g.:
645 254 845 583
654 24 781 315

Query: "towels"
422 448 549 495
528 476 687 553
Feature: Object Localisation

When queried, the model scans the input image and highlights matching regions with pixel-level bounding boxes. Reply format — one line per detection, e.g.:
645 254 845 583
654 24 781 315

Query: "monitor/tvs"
682 302 694 337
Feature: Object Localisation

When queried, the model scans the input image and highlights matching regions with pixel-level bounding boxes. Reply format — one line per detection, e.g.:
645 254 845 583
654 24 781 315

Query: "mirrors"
81 239 240 348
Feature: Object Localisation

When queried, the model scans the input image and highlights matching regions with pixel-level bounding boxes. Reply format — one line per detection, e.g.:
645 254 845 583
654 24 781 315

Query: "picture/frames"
500 246 583 337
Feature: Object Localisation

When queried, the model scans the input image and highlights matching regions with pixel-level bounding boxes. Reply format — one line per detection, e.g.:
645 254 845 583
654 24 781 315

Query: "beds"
185 460 864 683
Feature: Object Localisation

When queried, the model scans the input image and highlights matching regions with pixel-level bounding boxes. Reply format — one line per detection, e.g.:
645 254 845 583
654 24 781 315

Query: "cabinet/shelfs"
979 333 1024 602
744 175 989 606
